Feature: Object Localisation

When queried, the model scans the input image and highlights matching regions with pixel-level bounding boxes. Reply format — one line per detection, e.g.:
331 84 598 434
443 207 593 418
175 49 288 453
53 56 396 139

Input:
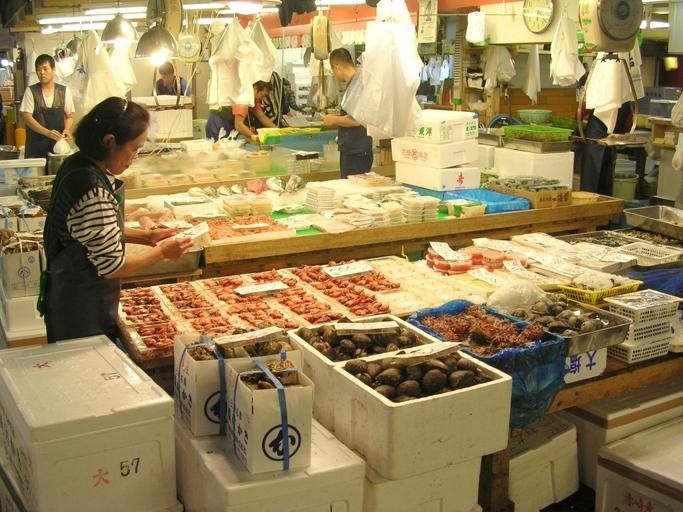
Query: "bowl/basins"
517 109 553 124
636 114 650 129
571 192 599 205
257 128 279 143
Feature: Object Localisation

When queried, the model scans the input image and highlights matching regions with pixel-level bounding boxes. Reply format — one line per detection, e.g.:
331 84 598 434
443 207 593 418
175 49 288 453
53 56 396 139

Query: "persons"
152 61 197 97
670 91 683 209
42 97 194 345
18 53 75 175
205 78 307 144
575 100 650 198
322 48 373 179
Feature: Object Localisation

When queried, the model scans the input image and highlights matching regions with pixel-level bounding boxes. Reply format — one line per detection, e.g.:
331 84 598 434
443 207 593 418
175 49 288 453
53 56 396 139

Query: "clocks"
522 0 554 34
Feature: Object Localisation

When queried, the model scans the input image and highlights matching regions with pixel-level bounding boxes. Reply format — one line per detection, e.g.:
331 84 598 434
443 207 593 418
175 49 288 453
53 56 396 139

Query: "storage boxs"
477 411 580 512
2 333 178 511
334 430 483 512
408 299 566 426
131 94 193 139
332 344 514 481
562 348 609 386
556 374 681 493
390 109 574 191
290 311 441 436
597 412 682 510
167 396 366 511
0 87 14 102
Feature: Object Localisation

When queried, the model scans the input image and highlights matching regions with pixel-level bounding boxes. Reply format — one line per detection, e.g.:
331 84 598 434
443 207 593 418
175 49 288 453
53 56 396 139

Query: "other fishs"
186 174 304 198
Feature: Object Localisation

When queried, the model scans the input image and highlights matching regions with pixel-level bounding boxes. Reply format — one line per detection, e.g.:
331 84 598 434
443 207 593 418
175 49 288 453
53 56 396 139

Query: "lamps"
34 0 329 66
639 13 669 29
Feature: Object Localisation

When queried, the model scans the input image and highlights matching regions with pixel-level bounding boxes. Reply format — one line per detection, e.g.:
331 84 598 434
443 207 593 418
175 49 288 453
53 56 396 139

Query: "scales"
176 34 201 108
304 0 342 114
569 0 649 147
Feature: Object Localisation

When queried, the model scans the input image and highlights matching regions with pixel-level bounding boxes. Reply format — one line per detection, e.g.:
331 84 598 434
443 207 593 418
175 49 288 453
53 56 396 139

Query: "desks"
124 165 624 287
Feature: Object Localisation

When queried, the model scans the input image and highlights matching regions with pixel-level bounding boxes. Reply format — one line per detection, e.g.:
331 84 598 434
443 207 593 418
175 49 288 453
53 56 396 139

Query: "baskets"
618 242 682 266
557 278 683 365
